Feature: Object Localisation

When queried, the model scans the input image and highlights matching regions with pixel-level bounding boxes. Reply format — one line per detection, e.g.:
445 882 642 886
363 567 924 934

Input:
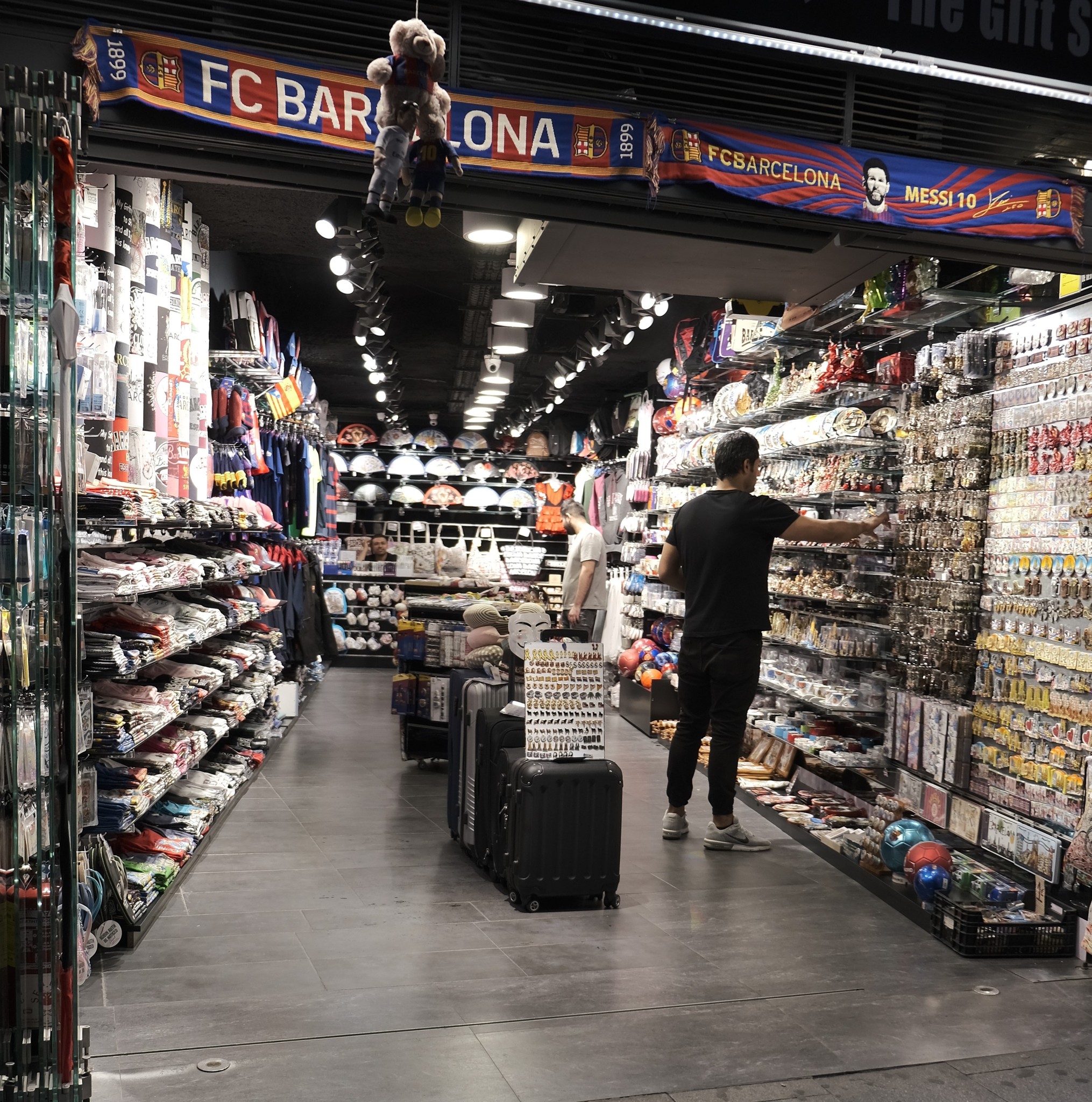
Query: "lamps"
316 198 407 431
463 210 517 245
463 266 673 438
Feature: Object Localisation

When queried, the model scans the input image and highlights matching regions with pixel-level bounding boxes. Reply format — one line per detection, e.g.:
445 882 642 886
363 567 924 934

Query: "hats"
210 383 253 491
497 435 524 452
209 496 284 530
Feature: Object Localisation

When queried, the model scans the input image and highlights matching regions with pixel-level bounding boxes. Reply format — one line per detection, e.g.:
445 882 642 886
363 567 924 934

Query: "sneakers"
704 816 772 852
661 807 689 839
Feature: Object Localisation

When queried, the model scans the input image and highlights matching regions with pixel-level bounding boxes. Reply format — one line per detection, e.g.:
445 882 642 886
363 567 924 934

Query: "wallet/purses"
345 584 408 651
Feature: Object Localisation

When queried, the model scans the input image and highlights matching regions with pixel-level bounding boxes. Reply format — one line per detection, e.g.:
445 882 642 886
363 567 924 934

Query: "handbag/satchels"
624 394 644 433
344 520 373 557
379 520 409 555
434 521 468 578
464 524 501 581
708 297 793 371
408 520 436 574
500 527 547 577
474 560 512 589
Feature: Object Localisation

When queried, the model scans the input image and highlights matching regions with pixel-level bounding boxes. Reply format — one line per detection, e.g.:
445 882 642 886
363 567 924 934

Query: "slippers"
73 833 138 985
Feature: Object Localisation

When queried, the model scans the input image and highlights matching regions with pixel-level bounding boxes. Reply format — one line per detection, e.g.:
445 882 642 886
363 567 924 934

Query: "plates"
759 675 885 710
652 381 897 474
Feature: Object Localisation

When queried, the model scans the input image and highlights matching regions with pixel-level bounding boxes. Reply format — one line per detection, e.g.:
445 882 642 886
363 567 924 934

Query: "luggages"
444 635 527 894
507 627 625 912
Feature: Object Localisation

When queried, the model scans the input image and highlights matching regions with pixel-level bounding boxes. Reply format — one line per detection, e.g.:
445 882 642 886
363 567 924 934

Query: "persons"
358 533 396 563
560 499 608 643
657 430 890 853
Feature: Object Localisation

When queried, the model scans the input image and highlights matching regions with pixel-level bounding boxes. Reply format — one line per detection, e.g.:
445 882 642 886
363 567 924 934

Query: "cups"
759 650 885 707
748 709 884 766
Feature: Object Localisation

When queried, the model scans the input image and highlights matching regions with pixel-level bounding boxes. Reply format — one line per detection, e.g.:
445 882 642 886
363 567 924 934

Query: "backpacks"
331 623 347 652
221 288 320 414
324 582 347 614
525 429 550 457
569 393 629 461
673 298 740 417
548 424 572 457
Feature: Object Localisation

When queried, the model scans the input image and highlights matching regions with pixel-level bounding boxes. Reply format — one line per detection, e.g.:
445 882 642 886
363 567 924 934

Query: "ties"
609 475 614 509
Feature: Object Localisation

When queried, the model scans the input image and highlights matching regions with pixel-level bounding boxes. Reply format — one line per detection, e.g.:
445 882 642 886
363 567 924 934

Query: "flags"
265 375 304 422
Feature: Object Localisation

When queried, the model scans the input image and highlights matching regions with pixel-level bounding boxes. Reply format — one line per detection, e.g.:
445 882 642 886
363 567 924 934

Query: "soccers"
879 820 936 874
904 840 953 883
913 864 951 903
617 637 679 688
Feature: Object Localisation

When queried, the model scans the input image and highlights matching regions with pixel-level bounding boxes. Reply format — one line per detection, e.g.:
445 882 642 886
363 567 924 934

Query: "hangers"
251 535 302 564
542 472 567 485
214 445 247 461
579 460 627 481
258 416 334 461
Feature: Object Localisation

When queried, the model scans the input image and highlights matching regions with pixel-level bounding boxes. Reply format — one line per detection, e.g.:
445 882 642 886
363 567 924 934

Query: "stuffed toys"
366 18 463 227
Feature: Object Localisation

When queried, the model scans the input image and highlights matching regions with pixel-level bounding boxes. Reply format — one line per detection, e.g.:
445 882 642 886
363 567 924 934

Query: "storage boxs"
935 891 1077 958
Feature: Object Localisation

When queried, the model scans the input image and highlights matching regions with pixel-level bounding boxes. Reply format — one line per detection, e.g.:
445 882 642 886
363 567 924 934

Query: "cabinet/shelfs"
315 448 583 659
14 165 298 951
385 595 557 768
595 298 1092 952
